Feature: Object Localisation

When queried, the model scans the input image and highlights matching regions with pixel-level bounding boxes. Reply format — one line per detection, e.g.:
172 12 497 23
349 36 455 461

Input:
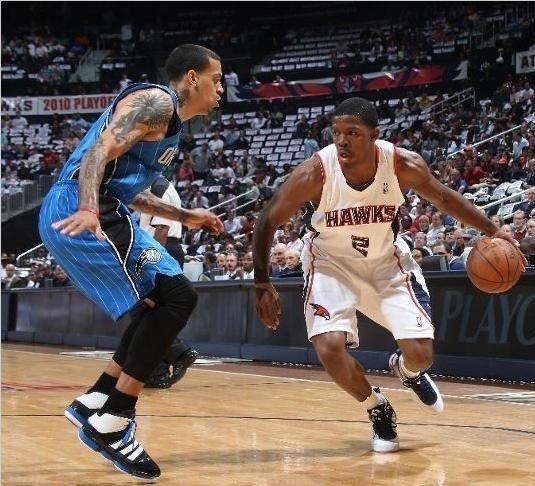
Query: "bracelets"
79 204 99 218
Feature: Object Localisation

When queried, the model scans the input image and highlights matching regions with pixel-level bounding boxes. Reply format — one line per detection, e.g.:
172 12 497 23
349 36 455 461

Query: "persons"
38 44 225 480
175 21 271 40
283 4 525 94
377 81 535 269
250 97 530 454
136 172 199 389
161 103 333 282
1 4 167 291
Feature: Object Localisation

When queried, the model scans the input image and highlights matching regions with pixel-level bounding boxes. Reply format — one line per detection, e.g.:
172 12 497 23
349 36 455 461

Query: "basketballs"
466 237 522 294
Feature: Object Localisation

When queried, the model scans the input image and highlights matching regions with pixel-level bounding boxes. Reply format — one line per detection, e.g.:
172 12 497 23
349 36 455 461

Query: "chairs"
1 14 535 192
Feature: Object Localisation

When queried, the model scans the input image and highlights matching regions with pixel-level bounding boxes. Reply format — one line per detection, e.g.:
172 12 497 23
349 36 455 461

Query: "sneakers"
144 375 169 389
167 350 197 386
367 389 400 453
65 392 161 480
388 351 443 416
